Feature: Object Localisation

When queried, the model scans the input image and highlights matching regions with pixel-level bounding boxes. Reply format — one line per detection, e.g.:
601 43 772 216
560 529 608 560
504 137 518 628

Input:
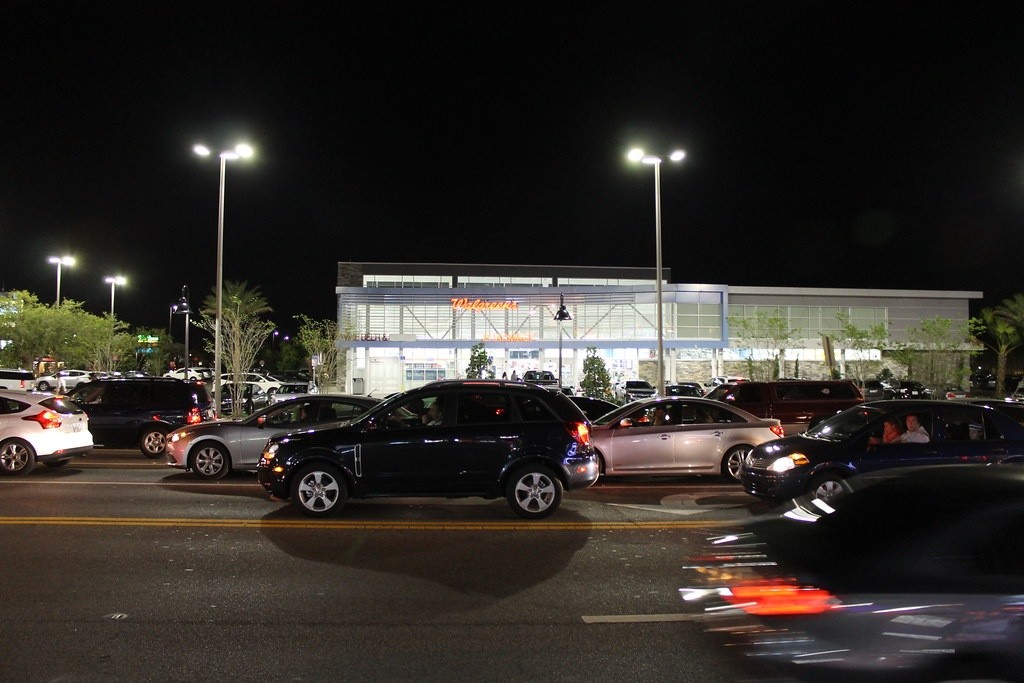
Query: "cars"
677 462 1024 683
739 400 1024 507
164 393 418 480
590 396 784 482
566 395 620 423
516 370 560 389
560 375 1024 405
1 366 317 416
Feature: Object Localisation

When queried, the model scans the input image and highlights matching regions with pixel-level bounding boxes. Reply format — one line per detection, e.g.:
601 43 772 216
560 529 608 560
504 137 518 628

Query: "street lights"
272 330 279 349
169 305 178 335
48 256 75 309
105 276 127 318
625 147 686 395
191 142 253 419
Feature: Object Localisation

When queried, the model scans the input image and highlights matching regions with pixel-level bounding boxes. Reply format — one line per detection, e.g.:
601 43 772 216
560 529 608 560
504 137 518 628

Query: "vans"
701 380 865 438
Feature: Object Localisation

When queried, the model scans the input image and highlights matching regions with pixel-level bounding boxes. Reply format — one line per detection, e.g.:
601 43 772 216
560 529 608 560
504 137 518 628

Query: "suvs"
61 377 219 459
256 379 600 519
0 389 95 475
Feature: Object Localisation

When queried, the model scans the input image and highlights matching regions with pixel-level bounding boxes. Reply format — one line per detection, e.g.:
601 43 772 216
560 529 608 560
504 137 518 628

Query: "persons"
871 421 901 443
296 406 311 423
631 404 666 426
511 370 519 381
390 402 442 427
88 372 98 380
899 414 930 442
968 421 983 439
692 407 712 424
502 372 508 380
53 373 67 395
